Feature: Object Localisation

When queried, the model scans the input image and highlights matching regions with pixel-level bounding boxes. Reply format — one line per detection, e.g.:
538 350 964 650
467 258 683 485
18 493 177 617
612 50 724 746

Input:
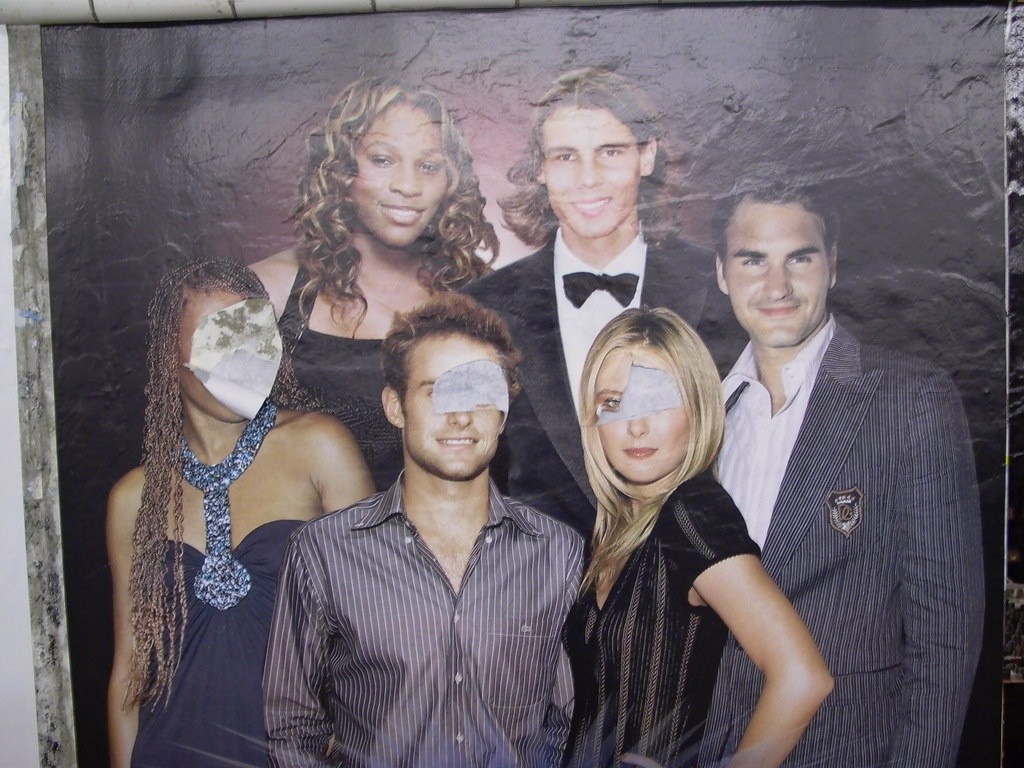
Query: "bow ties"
561 272 640 308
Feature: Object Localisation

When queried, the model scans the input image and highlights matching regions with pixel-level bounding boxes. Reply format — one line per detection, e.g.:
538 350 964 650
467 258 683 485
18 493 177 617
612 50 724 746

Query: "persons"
704 162 986 767
453 68 747 539
563 307 835 768
260 295 587 768
104 257 373 767
245 74 503 490
